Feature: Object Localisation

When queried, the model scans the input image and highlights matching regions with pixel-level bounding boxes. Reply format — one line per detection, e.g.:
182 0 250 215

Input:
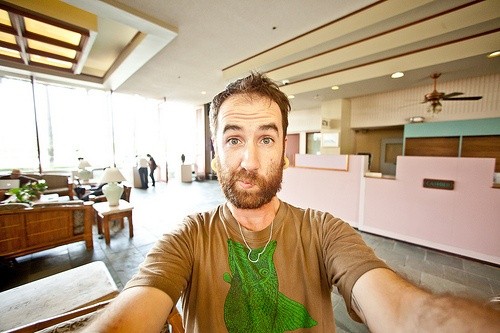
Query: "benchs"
0 200 94 262
0 169 73 200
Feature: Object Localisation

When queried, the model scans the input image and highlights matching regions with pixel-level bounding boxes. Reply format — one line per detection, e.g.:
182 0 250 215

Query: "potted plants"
8 179 48 206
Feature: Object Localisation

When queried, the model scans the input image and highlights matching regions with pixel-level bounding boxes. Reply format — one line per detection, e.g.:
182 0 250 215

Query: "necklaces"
233 208 277 263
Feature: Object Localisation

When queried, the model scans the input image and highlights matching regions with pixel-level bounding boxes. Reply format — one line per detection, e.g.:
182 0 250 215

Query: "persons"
134 155 148 190
147 153 158 187
79 70 500 333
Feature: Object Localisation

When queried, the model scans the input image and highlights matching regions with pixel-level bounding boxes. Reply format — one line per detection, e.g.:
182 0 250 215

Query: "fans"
419 72 483 104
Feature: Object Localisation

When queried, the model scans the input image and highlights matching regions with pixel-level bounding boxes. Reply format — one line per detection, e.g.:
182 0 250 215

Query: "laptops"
0 180 20 189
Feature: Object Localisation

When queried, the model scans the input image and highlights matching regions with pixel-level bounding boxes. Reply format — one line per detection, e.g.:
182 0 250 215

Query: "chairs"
1 297 185 333
89 185 132 225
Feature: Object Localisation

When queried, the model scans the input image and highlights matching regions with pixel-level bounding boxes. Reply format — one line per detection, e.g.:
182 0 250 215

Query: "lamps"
428 102 442 114
100 168 127 205
77 160 92 181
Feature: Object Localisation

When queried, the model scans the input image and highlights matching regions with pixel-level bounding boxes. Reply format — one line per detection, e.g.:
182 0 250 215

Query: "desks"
0 261 119 333
0 194 59 205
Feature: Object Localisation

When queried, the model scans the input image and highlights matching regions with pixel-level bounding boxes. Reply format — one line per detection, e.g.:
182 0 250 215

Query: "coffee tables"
93 199 134 245
77 180 97 186
